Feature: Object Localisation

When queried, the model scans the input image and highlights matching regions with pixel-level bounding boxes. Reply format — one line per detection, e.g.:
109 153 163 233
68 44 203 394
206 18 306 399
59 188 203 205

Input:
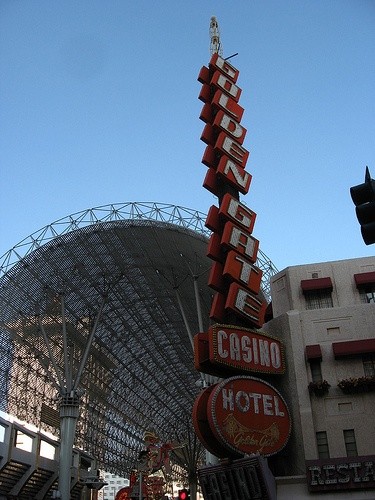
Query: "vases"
343 384 374 396
314 389 326 398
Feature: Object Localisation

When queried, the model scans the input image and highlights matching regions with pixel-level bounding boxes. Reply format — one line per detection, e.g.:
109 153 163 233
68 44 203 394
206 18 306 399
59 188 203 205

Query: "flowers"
307 380 330 397
336 375 374 394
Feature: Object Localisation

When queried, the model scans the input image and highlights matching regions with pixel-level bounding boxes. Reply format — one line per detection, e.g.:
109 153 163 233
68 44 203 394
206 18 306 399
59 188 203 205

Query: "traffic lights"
179 489 187 500
